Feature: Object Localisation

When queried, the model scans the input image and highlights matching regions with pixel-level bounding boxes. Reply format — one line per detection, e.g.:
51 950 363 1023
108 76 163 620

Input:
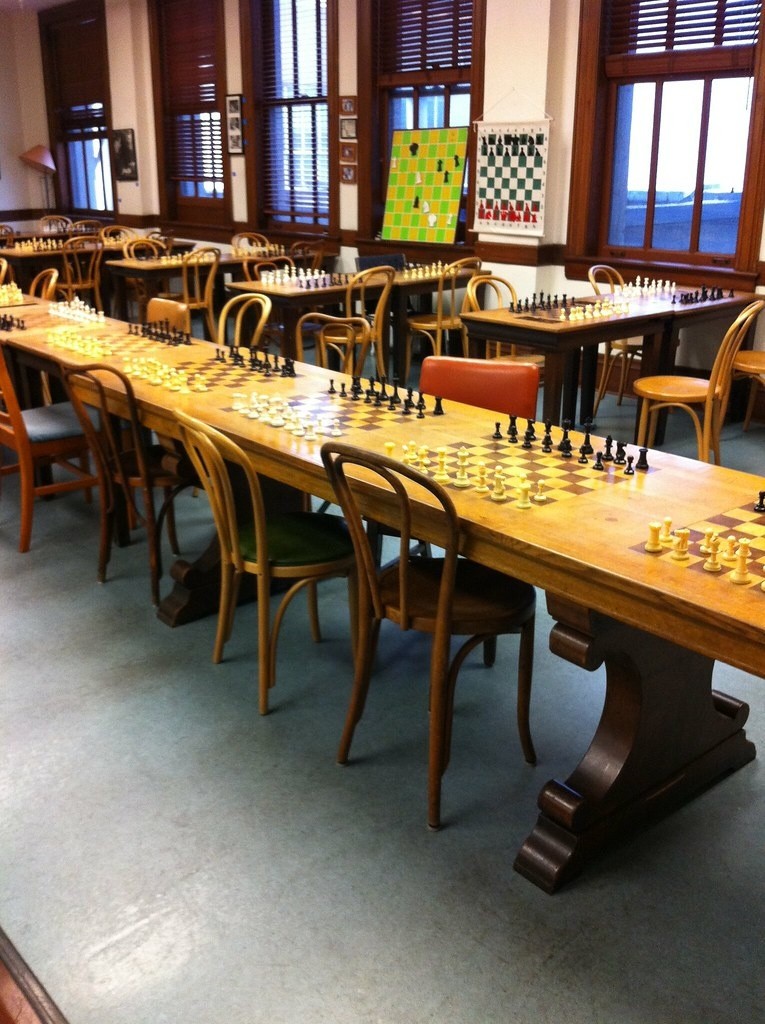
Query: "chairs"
0 207 765 833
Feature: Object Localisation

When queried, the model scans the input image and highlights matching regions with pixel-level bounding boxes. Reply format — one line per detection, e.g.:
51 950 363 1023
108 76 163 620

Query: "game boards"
382 127 469 244
628 290 736 306
514 301 628 322
119 353 307 396
475 121 549 231
627 491 765 598
216 388 450 445
0 295 38 310
0 314 108 339
258 276 367 292
47 331 191 357
377 429 663 516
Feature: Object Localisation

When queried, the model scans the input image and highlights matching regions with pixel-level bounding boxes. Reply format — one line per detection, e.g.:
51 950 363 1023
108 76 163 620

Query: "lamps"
20 143 56 229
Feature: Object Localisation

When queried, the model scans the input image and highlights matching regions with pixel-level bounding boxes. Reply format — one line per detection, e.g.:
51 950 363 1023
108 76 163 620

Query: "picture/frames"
226 94 244 155
111 129 138 182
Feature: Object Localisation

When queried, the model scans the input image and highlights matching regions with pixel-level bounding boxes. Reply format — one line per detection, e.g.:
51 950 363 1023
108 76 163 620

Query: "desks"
457 293 675 444
223 273 397 378
357 263 492 389
228 252 339 275
0 231 99 241
580 286 765 449
0 295 765 898
109 239 196 319
0 293 127 406
0 241 113 308
104 252 256 329
